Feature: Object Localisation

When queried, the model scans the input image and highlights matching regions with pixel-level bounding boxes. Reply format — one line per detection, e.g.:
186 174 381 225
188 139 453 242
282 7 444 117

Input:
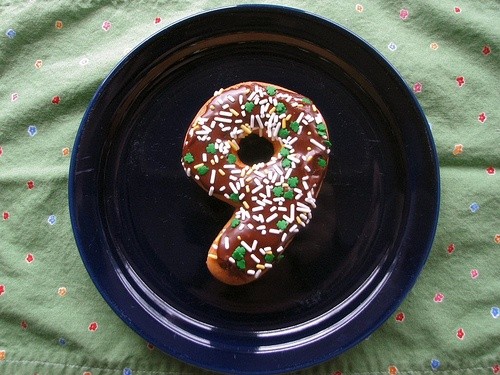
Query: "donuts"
181 82 331 286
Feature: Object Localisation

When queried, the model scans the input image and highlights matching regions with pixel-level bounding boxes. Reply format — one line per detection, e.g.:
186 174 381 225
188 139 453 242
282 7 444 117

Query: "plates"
68 2 441 375
